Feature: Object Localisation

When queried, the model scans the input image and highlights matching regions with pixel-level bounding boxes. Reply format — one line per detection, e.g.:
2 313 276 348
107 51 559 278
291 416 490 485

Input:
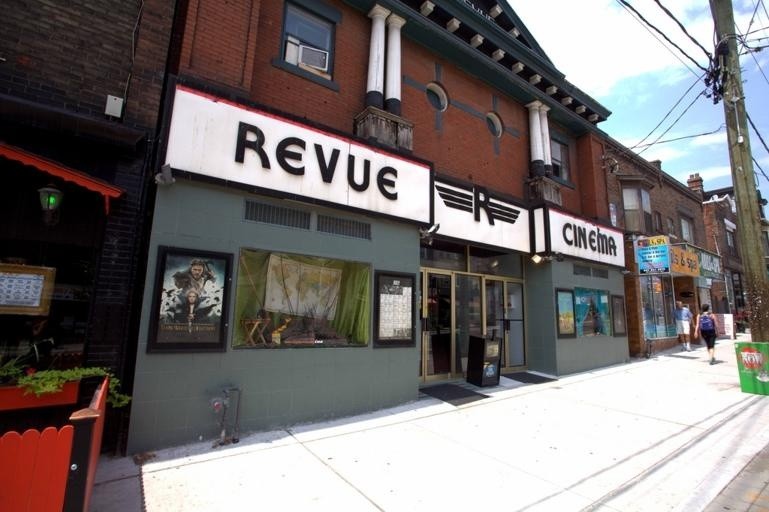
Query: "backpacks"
698 315 714 331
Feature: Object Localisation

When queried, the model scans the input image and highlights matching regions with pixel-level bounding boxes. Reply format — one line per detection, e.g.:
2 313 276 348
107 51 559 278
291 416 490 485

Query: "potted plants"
0 337 132 411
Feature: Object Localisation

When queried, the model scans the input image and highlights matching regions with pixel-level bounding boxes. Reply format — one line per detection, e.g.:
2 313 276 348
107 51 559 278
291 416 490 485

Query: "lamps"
419 223 440 239
530 254 552 265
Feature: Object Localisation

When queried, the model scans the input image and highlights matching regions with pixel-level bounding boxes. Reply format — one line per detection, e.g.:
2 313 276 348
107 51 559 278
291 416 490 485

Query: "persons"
694 304 720 365
670 301 696 351
173 259 217 324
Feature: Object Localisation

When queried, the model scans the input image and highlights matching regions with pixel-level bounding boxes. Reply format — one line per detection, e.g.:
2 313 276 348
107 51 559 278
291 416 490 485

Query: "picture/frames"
0 262 56 316
145 244 235 354
373 269 417 348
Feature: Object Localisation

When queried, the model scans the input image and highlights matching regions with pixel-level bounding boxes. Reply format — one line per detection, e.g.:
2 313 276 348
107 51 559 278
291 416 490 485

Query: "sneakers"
709 358 718 366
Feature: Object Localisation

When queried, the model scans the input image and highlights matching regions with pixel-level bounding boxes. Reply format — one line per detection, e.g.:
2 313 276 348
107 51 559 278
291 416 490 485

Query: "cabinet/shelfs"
465 334 503 387
431 333 462 375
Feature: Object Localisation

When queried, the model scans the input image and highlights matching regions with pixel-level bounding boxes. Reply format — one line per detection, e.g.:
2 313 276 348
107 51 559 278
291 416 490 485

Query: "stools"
240 318 270 348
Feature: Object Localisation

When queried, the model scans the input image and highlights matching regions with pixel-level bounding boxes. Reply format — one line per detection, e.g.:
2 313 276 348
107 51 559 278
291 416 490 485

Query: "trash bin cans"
734 342 769 396
